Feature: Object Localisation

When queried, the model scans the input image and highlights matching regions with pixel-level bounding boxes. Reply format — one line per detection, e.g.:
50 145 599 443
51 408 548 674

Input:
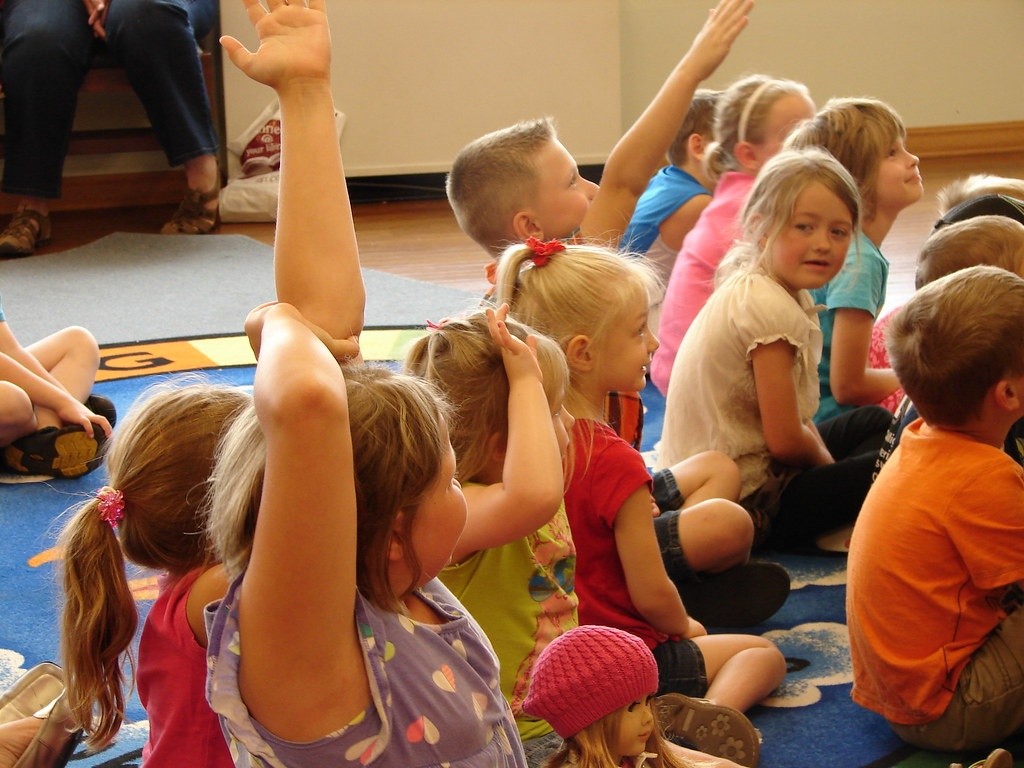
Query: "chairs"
0 0 229 217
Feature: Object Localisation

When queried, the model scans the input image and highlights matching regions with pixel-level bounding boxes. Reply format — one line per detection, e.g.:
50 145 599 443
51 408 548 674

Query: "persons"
0 1 223 260
0 304 117 478
59 0 1024 768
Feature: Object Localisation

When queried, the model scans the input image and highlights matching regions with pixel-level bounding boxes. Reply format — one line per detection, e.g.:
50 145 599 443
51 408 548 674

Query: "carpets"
0 230 931 768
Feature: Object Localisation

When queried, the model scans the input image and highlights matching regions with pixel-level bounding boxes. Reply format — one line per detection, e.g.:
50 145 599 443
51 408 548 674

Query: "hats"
520 623 658 739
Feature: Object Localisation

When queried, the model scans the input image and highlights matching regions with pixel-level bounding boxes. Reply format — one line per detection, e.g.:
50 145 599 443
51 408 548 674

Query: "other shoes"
967 748 1013 768
0 662 68 731
13 675 91 768
85 394 117 435
654 692 758 767
677 563 790 629
0 423 109 478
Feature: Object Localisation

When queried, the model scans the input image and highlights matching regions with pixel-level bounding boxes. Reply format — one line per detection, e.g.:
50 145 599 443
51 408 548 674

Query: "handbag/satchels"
219 96 347 220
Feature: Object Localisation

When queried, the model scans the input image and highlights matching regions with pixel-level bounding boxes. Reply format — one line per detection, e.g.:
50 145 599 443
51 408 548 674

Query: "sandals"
0 206 48 255
160 159 221 236
